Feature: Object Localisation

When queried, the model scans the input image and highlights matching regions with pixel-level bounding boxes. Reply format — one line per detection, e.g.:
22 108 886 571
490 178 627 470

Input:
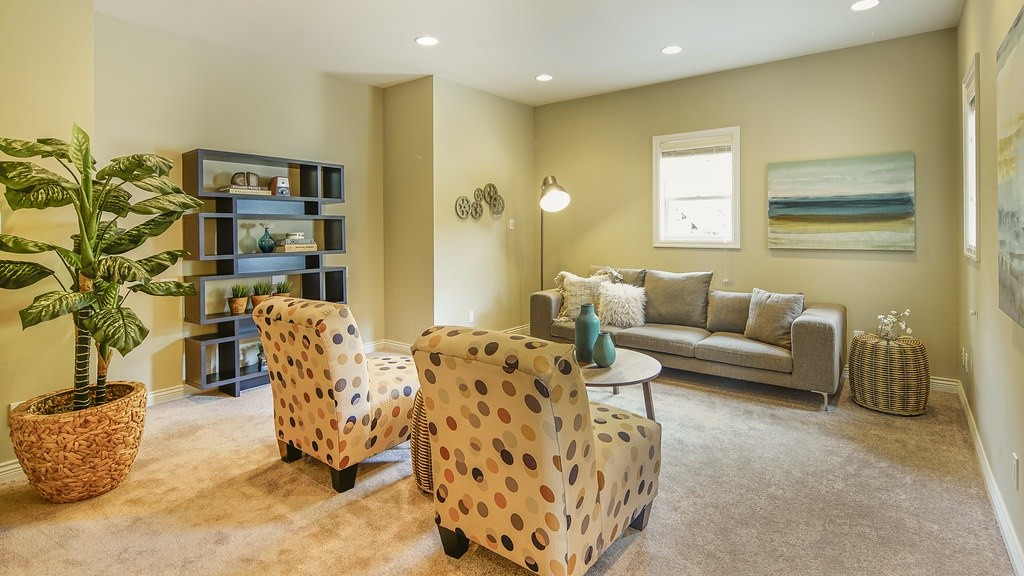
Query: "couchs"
252 295 422 494
410 325 661 576
530 266 847 412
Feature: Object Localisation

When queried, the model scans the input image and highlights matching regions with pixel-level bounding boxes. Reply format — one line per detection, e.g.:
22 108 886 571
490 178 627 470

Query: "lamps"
540 174 570 292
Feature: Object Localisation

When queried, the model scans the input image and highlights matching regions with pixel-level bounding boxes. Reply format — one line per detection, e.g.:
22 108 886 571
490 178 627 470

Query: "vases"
575 302 601 363
591 332 617 369
257 228 278 253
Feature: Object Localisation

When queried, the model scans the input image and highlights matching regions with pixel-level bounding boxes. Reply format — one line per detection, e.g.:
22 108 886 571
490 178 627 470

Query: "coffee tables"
570 345 662 422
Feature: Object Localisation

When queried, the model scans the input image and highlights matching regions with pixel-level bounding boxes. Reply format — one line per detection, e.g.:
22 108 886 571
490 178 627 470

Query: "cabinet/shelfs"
182 148 348 398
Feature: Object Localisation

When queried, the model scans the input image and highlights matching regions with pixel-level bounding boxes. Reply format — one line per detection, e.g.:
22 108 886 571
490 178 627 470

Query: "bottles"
575 304 600 363
593 333 616 368
258 228 276 253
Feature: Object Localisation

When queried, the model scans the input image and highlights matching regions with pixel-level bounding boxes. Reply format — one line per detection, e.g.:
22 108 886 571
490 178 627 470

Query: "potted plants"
251 282 276 310
227 283 252 316
273 280 295 297
254 342 268 373
0 125 207 506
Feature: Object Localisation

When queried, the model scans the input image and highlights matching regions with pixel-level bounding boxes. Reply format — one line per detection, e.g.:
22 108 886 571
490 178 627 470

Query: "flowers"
871 305 917 334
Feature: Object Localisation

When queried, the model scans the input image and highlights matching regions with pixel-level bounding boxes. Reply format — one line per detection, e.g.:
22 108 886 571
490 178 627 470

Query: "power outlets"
510 219 514 230
468 311 473 323
1011 452 1018 490
853 330 864 338
960 347 968 373
9 401 25 427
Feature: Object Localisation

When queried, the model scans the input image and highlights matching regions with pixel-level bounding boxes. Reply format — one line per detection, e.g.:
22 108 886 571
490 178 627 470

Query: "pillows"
639 268 715 329
554 267 624 323
704 290 754 333
598 282 648 327
743 286 803 351
609 267 647 287
